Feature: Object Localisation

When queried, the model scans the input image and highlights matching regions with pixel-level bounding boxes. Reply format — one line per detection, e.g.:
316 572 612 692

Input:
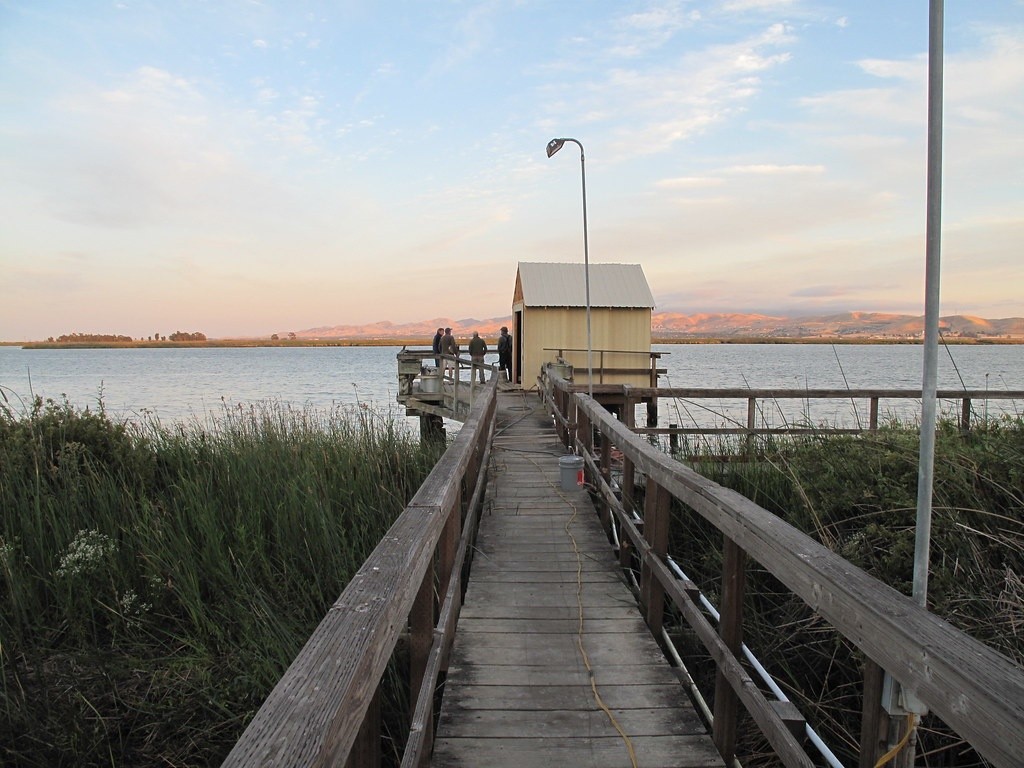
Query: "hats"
500 327 508 332
444 328 452 333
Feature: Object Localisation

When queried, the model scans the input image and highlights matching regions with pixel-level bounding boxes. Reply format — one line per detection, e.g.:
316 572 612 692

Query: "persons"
433 328 444 366
498 327 512 381
438 328 460 385
469 331 486 384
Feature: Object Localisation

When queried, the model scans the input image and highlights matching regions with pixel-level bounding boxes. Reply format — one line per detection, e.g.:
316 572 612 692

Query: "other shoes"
479 381 486 384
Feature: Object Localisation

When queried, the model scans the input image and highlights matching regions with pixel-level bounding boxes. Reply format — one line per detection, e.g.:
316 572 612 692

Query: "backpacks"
500 335 512 356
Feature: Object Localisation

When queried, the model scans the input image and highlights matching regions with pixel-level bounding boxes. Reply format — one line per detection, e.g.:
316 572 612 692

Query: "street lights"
545 137 593 399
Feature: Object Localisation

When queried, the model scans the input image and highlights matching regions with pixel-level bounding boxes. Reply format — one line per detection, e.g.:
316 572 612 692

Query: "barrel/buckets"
559 455 585 492
420 366 439 393
